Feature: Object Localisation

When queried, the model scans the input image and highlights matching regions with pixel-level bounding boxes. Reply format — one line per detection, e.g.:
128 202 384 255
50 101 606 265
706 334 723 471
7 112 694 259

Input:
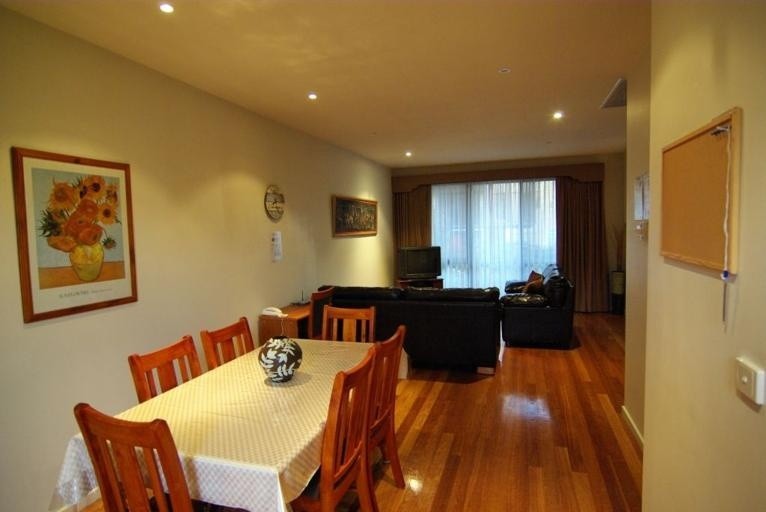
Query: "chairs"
502 264 574 348
365 325 405 512
128 335 204 404
322 305 375 342
74 403 223 512
289 347 375 512
200 316 254 371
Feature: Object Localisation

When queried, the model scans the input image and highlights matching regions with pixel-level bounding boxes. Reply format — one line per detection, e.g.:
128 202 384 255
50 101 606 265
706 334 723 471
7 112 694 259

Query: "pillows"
505 268 547 306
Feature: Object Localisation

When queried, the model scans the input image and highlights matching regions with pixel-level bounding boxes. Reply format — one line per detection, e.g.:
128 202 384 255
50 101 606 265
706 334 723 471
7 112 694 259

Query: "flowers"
38 173 122 253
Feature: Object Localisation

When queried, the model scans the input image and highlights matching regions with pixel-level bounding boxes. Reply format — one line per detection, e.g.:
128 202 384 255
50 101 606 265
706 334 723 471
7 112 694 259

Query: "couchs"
298 284 501 374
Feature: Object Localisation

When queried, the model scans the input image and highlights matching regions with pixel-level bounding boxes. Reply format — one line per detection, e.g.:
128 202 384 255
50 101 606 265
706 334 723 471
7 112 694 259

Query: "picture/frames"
332 195 378 237
10 146 138 324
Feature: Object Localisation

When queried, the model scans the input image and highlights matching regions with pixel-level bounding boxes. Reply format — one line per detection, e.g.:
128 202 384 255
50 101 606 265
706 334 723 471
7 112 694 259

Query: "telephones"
262 307 282 317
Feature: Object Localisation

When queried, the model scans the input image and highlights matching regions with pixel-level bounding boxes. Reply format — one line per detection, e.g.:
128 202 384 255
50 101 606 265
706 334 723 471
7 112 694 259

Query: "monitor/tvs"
399 246 442 281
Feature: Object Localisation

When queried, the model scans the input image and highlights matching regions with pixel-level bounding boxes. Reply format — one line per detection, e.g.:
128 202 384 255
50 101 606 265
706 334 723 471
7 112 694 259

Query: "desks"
260 303 310 344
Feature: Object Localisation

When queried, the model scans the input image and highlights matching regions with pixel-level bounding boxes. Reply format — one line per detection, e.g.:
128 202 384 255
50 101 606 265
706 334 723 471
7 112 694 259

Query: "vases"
612 270 625 315
258 336 302 383
69 243 105 281
